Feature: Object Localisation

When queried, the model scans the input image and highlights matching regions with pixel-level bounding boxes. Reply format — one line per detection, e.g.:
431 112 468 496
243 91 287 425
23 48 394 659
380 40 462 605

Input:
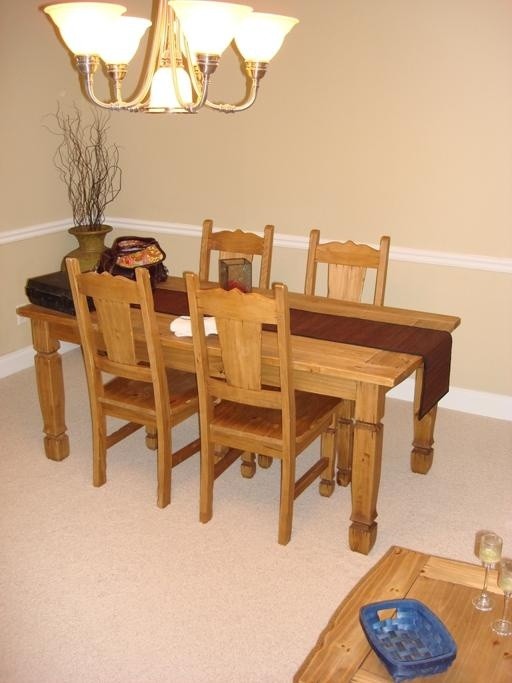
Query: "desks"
293 544 512 682
15 274 461 556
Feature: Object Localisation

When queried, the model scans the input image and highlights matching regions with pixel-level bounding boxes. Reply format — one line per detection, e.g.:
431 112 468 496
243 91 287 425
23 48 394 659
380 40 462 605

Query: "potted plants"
42 96 123 275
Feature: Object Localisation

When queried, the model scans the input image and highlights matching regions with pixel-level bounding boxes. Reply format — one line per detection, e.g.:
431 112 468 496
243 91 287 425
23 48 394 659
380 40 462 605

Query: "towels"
170 314 219 337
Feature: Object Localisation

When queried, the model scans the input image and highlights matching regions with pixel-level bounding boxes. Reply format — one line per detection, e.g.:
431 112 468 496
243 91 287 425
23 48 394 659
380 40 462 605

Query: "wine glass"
473 531 512 638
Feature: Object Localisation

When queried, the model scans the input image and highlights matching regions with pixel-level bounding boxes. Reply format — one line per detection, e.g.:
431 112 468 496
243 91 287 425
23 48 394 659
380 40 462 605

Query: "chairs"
258 229 391 488
199 219 274 288
65 258 229 509
185 272 344 546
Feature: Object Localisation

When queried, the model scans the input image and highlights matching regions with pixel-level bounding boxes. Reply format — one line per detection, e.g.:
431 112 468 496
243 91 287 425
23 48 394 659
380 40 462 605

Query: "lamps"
43 0 299 115
